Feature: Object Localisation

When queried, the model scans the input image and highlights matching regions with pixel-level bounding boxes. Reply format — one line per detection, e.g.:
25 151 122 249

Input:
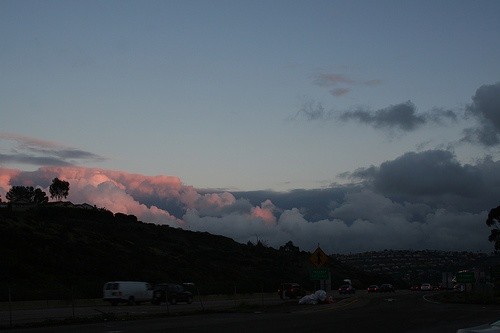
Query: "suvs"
152 284 195 304
278 283 305 299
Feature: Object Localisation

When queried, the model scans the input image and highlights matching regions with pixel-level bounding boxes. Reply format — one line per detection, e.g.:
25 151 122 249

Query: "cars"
380 284 392 292
338 284 355 295
367 285 379 292
420 283 433 291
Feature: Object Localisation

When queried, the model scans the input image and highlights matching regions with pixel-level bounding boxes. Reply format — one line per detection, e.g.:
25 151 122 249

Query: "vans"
103 281 154 305
